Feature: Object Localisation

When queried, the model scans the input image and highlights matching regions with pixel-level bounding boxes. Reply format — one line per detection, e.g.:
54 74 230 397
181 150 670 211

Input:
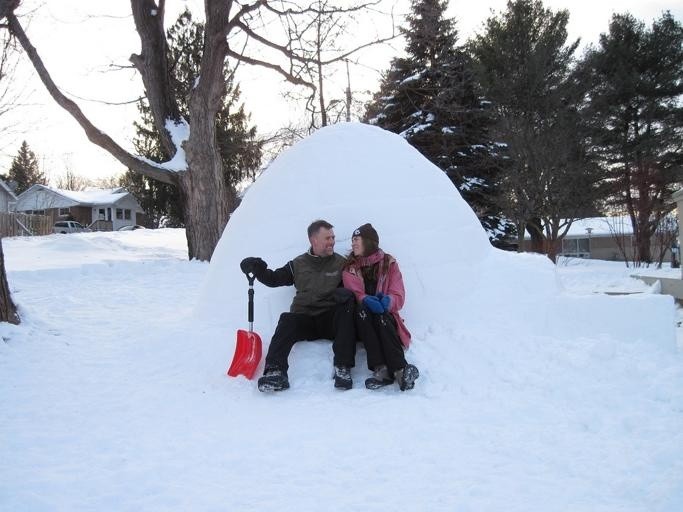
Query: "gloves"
240 257 268 276
332 288 352 304
362 295 392 313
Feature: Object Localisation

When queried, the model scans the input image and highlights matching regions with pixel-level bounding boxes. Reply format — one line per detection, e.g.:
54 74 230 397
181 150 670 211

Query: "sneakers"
258 370 289 393
334 366 352 390
365 358 419 391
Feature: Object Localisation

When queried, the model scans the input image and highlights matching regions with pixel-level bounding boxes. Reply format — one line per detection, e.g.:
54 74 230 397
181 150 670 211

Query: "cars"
117 225 146 231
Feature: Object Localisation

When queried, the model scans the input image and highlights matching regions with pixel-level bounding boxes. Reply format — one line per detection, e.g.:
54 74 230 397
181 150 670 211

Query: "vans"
52 220 92 234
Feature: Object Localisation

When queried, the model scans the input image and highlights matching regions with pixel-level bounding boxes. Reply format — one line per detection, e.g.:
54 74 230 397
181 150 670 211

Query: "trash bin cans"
671 247 680 268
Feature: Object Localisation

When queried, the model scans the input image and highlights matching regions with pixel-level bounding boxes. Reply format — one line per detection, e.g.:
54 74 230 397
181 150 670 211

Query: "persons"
241 221 356 392
342 223 419 391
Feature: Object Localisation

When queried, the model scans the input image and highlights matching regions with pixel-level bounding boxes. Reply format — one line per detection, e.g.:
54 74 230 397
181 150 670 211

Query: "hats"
352 223 379 243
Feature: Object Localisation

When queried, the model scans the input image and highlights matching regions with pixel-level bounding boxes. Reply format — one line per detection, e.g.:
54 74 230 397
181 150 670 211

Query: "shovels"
227 271 263 380
